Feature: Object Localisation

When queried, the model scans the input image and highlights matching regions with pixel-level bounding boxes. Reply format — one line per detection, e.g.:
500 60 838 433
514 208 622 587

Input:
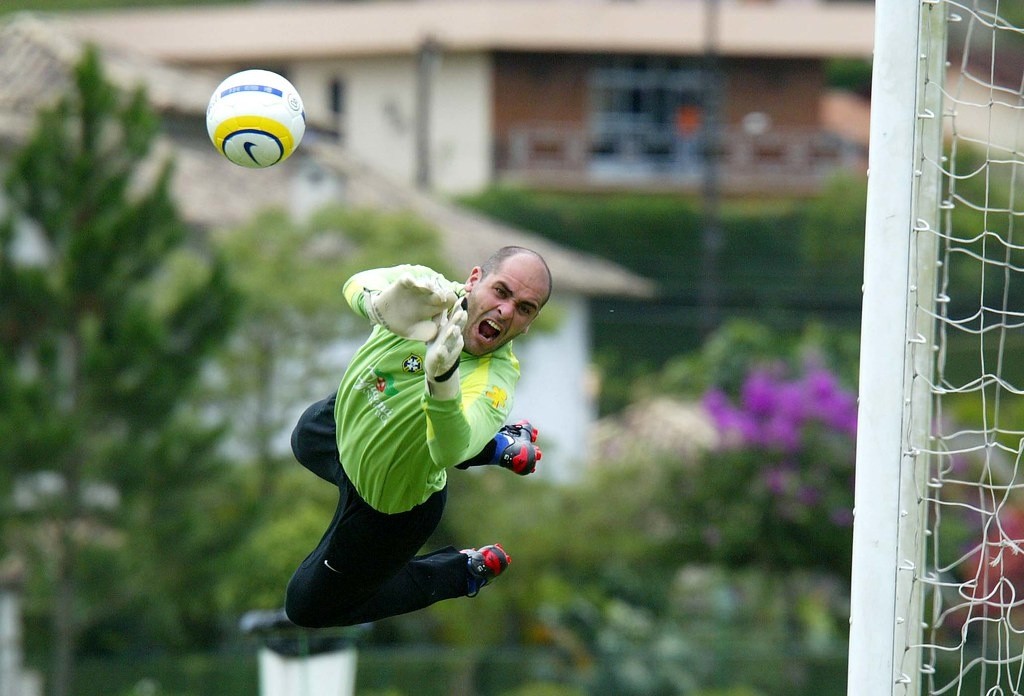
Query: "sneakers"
459 544 512 598
499 419 542 476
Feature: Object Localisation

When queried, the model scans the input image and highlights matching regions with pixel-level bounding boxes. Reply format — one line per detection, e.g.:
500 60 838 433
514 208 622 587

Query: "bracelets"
434 355 461 383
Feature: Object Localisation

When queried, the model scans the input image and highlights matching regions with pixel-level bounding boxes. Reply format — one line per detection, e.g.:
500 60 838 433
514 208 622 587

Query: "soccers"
206 69 306 170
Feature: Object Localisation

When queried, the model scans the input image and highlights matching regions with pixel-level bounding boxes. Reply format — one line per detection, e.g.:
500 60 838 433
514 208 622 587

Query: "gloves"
363 272 457 341
424 296 469 400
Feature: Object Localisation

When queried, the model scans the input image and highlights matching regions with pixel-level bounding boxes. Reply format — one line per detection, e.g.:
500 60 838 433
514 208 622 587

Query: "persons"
284 245 554 629
954 458 1024 632
